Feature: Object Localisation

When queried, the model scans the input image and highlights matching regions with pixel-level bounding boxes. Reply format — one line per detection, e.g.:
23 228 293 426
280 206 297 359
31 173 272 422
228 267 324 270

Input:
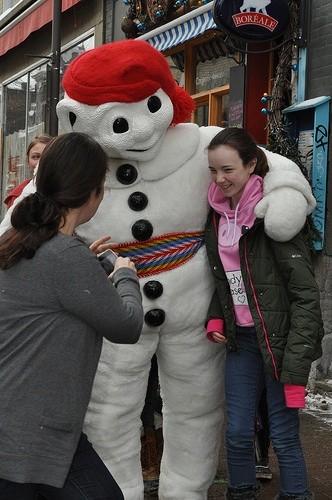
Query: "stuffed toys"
0 38 318 500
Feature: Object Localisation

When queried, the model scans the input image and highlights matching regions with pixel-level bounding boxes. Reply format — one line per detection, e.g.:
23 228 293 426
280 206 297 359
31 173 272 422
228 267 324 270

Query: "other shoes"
256 467 274 479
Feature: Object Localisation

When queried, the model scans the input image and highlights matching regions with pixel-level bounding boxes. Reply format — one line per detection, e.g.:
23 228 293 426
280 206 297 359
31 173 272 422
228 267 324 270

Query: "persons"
3 131 57 213
202 127 326 500
0 132 145 500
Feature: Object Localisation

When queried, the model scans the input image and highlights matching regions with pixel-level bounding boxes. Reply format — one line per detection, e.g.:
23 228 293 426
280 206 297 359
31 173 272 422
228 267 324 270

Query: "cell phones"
96 249 119 278
